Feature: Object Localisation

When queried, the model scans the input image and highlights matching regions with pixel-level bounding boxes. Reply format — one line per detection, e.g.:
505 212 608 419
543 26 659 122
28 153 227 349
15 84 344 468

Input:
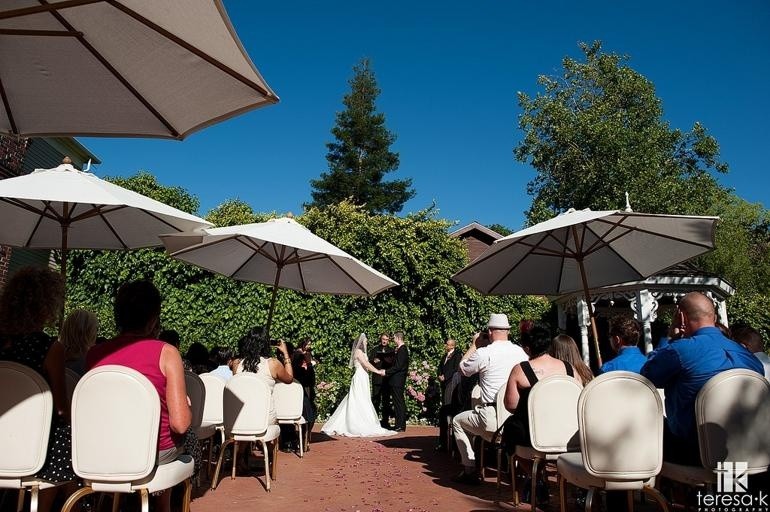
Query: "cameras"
271 340 280 346
480 332 489 341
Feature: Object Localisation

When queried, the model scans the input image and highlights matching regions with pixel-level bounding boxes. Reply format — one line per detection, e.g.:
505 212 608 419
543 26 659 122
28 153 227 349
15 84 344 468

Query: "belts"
483 403 496 406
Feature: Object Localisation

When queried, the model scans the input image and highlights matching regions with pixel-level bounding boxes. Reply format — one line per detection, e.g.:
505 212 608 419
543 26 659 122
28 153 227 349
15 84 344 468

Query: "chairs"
1 358 309 512
442 366 770 512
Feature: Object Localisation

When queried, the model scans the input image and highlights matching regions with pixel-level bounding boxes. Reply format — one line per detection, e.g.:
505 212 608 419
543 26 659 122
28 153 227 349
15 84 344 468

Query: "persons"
320 333 398 437
383 331 409 431
436 293 769 501
369 333 394 430
0 269 318 512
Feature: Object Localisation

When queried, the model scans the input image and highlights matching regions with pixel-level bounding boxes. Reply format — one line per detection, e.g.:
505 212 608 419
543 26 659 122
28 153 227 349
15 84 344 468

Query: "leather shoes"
522 477 549 503
381 420 405 431
282 442 310 452
451 469 481 483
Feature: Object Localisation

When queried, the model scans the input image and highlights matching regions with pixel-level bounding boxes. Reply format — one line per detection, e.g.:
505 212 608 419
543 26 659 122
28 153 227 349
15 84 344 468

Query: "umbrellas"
448 207 719 367
0 0 279 142
1 156 215 339
157 210 401 339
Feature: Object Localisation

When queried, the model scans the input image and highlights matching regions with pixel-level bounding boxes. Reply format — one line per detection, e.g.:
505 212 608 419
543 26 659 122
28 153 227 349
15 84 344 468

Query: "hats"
485 314 511 329
